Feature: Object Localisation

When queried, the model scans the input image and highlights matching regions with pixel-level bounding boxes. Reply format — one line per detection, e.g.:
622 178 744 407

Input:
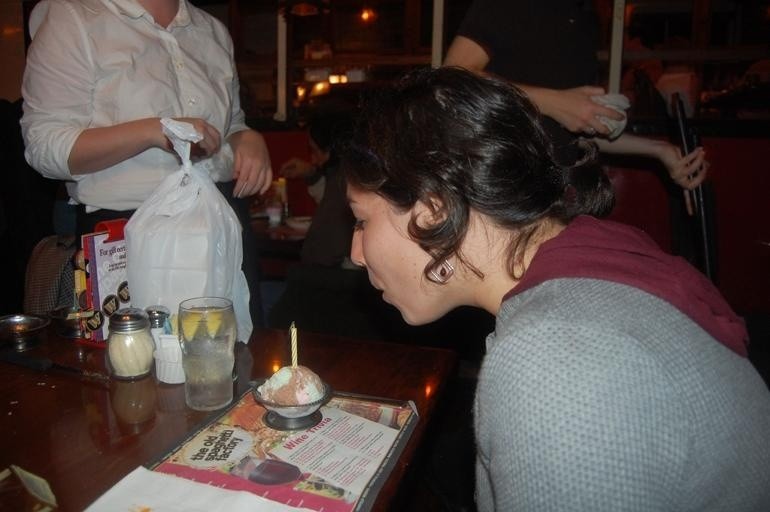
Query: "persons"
259 102 356 329
437 0 712 217
19 0 275 329
328 63 769 510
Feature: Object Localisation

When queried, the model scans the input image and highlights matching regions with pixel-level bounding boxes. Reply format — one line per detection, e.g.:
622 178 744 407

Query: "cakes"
257 364 325 419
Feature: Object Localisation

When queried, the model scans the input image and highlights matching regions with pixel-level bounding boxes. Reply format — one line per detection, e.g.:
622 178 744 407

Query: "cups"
177 295 237 412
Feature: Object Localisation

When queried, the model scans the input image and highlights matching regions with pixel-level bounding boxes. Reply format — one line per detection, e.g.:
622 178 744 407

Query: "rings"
582 126 595 137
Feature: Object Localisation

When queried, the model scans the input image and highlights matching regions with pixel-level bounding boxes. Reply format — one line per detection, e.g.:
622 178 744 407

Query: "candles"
291 327 298 366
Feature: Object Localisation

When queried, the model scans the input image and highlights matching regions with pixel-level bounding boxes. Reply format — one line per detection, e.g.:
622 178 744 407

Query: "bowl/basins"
252 378 332 420
0 313 53 353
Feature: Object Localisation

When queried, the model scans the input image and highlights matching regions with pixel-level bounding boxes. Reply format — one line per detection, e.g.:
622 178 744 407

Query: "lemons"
206 307 225 338
181 307 203 342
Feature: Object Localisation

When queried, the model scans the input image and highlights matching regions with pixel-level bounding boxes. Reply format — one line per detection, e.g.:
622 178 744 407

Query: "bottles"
105 304 186 384
266 174 290 227
